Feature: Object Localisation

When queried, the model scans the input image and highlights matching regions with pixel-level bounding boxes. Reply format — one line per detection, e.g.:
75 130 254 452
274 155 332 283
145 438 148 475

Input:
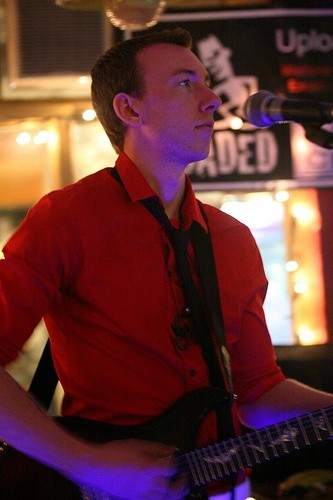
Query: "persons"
0 27 333 500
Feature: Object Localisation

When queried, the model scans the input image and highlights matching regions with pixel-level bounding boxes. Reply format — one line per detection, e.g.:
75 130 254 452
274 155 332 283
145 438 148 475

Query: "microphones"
245 91 333 128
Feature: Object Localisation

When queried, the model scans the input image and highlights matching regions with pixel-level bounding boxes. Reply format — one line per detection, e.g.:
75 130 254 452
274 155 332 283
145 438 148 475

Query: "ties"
169 230 238 494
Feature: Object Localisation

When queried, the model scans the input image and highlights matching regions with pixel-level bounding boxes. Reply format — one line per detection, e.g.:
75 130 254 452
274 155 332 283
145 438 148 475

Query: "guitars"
0 386 333 500
